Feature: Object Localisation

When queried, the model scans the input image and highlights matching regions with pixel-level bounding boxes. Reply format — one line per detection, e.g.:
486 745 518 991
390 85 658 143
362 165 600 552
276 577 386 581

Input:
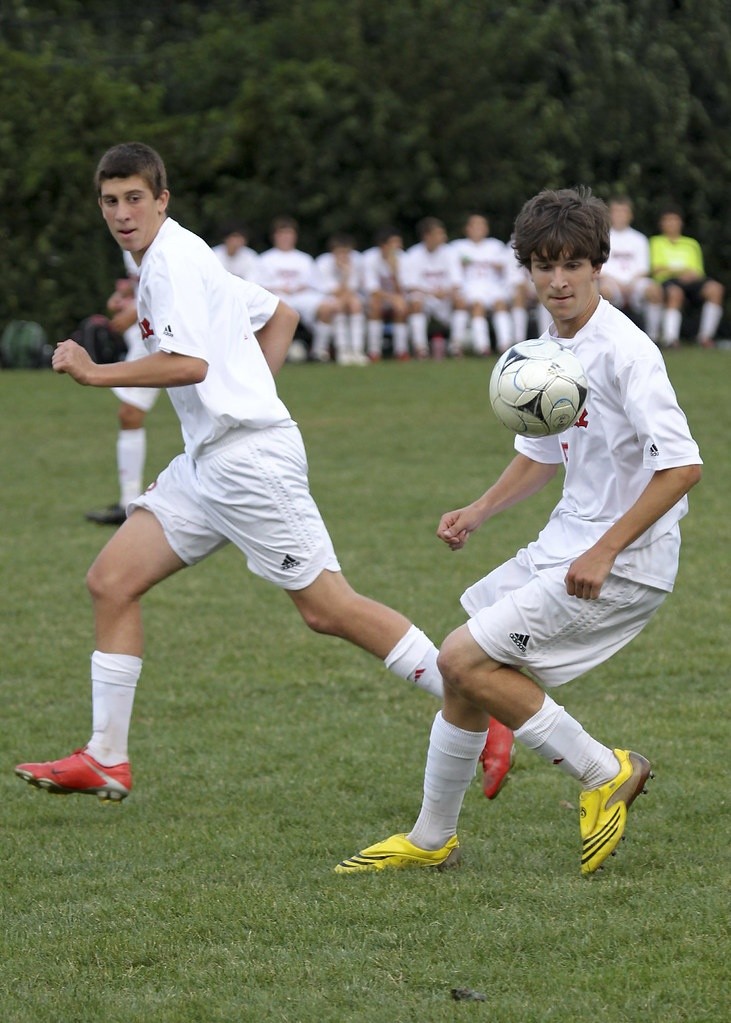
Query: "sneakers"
15 745 132 802
333 833 460 874
578 748 655 875
480 715 517 800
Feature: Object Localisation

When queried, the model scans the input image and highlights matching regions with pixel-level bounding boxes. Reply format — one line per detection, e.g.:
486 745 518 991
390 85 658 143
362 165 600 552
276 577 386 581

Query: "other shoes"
85 505 127 525
287 337 717 366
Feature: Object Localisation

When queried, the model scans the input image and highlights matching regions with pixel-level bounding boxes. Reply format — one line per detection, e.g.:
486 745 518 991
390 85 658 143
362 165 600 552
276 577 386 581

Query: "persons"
84 189 722 526
11 164 518 802
334 190 702 880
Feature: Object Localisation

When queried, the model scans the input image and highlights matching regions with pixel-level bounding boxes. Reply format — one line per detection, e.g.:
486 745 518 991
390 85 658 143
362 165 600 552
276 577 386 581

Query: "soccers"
488 337 591 440
